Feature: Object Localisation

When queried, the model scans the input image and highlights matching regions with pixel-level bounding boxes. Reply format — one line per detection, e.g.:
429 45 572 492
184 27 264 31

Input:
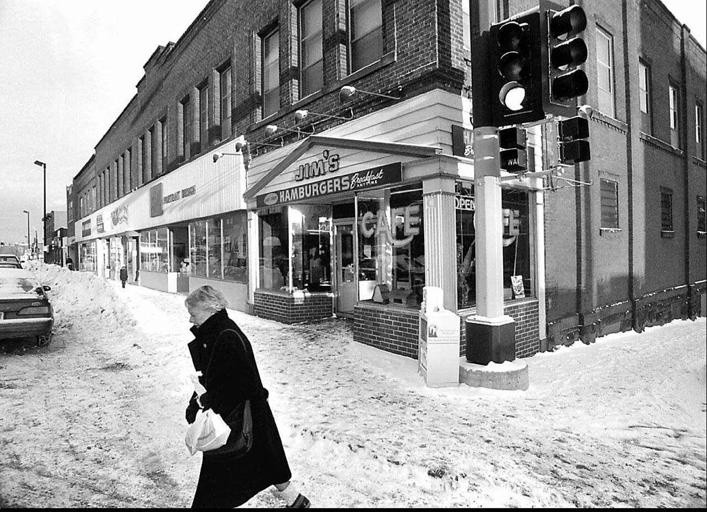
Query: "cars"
139 244 283 286
0 254 24 268
344 256 426 297
0 267 54 346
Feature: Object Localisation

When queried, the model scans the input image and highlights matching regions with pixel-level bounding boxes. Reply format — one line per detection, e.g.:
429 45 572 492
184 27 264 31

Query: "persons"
119 266 128 288
184 284 312 509
66 255 73 270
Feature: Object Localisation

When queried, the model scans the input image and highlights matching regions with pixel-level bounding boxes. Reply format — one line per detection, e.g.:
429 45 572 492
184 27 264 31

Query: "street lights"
34 159 47 263
23 210 30 260
34 230 39 258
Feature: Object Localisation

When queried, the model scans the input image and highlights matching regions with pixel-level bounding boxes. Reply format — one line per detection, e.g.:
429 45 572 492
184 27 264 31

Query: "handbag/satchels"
201 396 256 464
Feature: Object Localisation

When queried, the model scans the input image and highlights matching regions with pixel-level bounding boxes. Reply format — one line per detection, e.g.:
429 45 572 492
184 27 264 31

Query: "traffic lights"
560 117 590 163
497 128 528 174
538 1 589 120
488 11 544 128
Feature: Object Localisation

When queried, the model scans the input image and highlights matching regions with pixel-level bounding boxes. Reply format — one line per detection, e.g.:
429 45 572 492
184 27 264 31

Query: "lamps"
210 82 404 164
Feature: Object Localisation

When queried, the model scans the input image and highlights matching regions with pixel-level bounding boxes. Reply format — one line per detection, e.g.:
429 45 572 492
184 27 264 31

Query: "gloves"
185 398 203 424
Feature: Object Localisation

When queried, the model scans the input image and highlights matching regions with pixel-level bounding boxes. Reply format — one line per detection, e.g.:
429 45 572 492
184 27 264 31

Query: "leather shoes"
286 492 312 508
